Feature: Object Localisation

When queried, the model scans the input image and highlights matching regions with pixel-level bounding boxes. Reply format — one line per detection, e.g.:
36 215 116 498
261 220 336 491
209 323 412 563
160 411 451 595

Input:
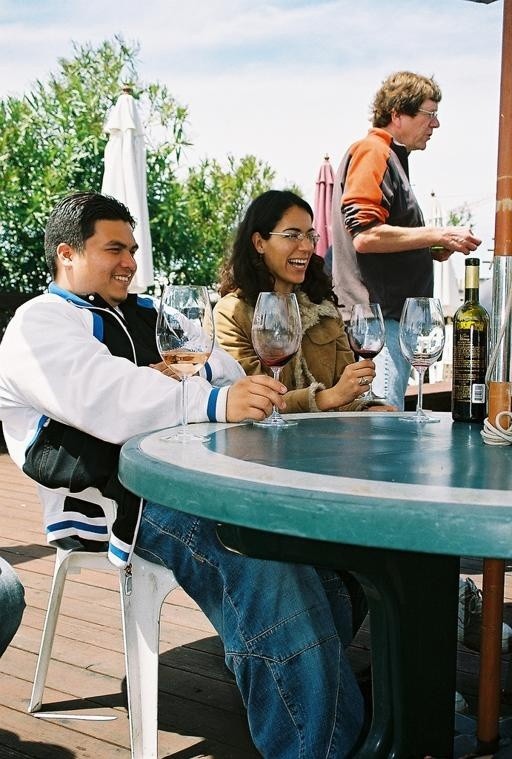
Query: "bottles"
452 257 490 424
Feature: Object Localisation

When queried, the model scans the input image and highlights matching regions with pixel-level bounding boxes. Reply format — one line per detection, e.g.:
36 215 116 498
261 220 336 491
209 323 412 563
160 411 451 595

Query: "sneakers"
458 576 512 655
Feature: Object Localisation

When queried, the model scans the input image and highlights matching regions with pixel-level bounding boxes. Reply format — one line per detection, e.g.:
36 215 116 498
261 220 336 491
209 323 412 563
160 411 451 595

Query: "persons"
323 73 481 414
196 190 512 717
0 192 386 759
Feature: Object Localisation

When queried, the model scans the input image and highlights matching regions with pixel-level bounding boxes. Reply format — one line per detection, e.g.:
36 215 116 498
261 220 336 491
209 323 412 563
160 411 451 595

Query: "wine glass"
246 287 301 430
397 295 447 424
347 301 388 402
151 281 219 446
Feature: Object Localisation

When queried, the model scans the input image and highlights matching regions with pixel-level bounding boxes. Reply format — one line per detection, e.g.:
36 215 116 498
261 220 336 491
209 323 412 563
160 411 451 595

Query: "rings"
358 377 368 385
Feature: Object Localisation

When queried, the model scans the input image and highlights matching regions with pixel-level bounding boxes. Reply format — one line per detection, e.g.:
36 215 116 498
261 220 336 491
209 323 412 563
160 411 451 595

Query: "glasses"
269 230 320 244
418 109 438 119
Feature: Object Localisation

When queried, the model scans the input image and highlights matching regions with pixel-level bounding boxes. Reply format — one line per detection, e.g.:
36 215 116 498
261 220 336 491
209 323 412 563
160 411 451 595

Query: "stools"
27 548 185 759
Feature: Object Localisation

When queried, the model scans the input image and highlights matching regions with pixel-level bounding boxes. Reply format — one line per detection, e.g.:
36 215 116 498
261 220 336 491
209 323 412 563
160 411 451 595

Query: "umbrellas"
98 66 156 302
314 154 340 260
424 190 450 311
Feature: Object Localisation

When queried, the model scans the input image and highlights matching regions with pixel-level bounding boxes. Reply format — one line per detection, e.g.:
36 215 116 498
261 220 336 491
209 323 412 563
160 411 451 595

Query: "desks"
116 403 510 758
